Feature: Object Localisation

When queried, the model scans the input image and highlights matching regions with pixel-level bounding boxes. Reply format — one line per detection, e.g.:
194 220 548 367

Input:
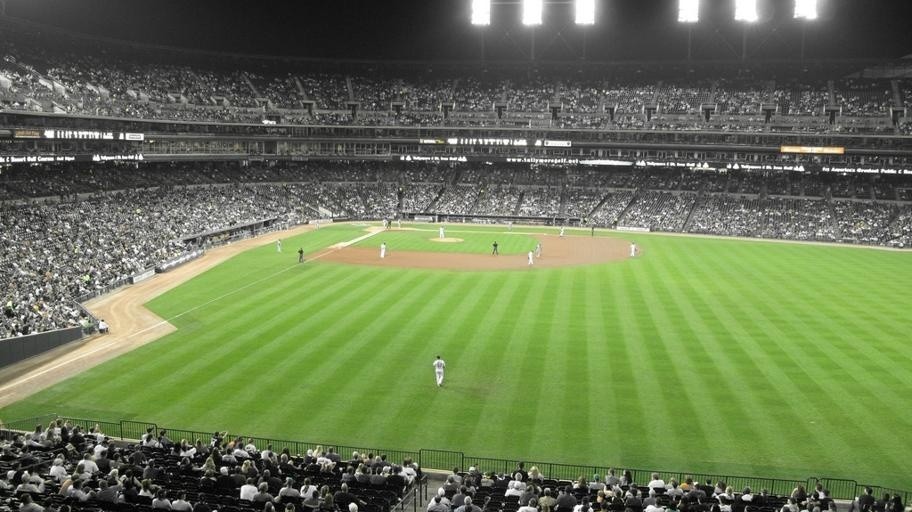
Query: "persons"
427 462 905 512
380 242 386 259
297 247 304 263
432 355 446 387
276 239 281 251
0 238 206 338
528 250 535 265
536 242 543 256
491 240 498 256
630 241 636 256
1 25 911 246
0 417 423 512
